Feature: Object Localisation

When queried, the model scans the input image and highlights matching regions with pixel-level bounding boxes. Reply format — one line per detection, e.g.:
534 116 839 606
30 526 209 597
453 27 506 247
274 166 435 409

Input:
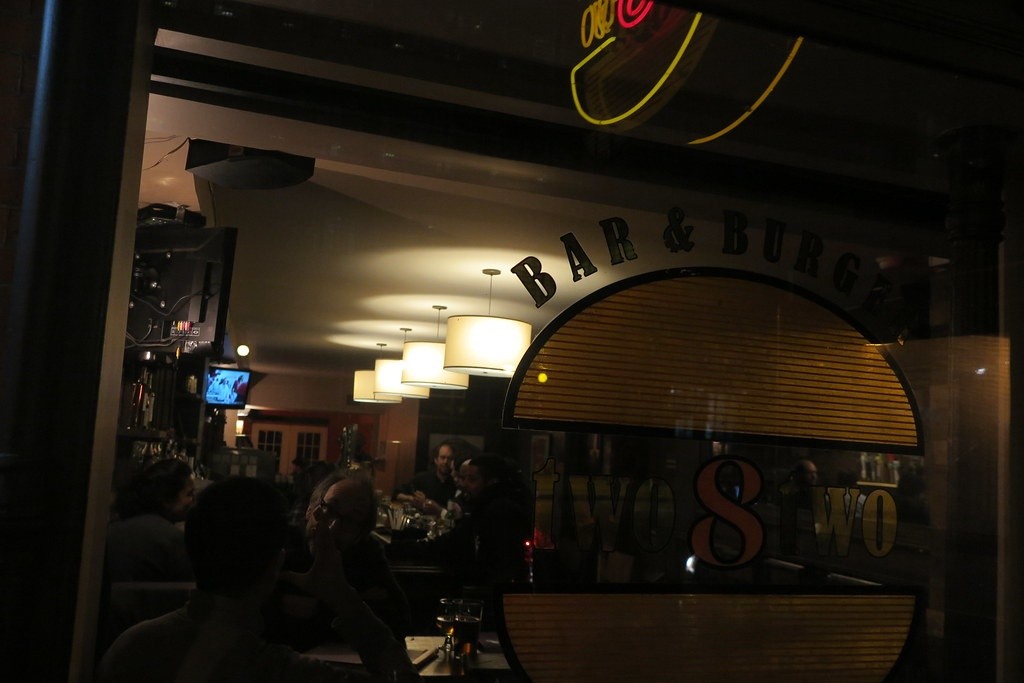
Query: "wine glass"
449 599 483 675
436 597 463 651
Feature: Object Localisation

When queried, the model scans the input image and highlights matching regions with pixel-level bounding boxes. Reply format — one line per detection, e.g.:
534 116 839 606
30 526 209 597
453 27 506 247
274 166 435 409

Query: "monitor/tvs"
205 366 251 410
126 227 239 355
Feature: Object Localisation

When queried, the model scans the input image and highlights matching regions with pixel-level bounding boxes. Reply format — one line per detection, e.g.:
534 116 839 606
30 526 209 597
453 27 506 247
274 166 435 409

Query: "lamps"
352 343 403 404
401 305 469 390
373 328 430 398
443 268 533 378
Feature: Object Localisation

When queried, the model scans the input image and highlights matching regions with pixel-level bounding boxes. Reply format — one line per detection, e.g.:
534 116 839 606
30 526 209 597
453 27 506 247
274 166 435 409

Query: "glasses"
320 497 349 518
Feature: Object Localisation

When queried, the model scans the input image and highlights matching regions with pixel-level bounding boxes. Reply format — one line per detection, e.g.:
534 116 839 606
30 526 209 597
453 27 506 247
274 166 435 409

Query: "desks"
300 634 521 683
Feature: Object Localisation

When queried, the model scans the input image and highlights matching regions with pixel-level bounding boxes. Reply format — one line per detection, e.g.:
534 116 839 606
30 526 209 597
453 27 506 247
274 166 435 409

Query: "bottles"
374 489 389 519
131 440 189 467
445 499 458 527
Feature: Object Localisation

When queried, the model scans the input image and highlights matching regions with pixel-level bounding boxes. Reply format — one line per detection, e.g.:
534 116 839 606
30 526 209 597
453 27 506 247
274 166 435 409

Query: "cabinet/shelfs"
107 349 210 530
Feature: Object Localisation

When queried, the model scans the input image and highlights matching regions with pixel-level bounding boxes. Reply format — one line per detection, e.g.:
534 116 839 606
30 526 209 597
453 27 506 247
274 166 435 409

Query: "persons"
792 457 818 509
98 457 422 683
390 443 534 606
